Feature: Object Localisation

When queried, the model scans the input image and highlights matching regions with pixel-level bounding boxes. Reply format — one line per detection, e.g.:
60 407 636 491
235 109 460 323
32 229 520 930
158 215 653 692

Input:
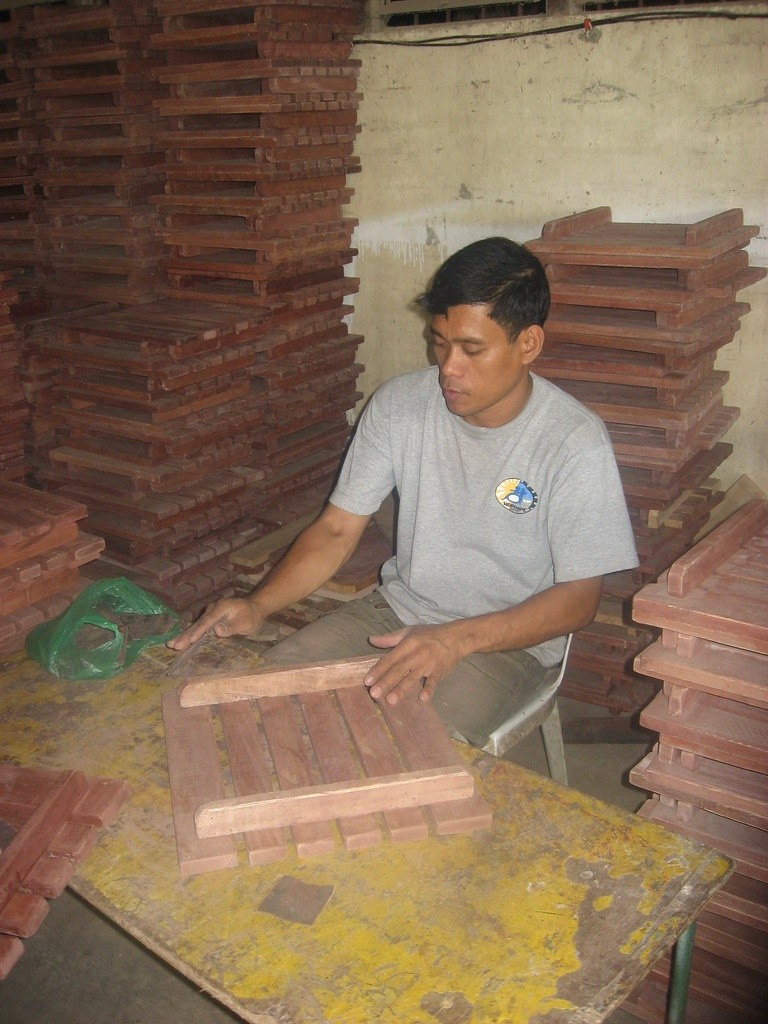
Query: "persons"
165 237 640 749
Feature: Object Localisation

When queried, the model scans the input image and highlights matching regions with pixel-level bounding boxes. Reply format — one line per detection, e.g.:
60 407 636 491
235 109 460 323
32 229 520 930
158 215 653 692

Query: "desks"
0 617 737 1024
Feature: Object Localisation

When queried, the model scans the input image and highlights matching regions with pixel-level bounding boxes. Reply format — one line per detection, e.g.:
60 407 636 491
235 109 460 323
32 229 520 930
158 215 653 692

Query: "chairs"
484 632 573 787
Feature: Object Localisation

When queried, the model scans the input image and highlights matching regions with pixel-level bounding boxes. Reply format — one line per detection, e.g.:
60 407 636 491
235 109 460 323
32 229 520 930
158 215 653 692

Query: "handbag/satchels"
23 570 184 682
